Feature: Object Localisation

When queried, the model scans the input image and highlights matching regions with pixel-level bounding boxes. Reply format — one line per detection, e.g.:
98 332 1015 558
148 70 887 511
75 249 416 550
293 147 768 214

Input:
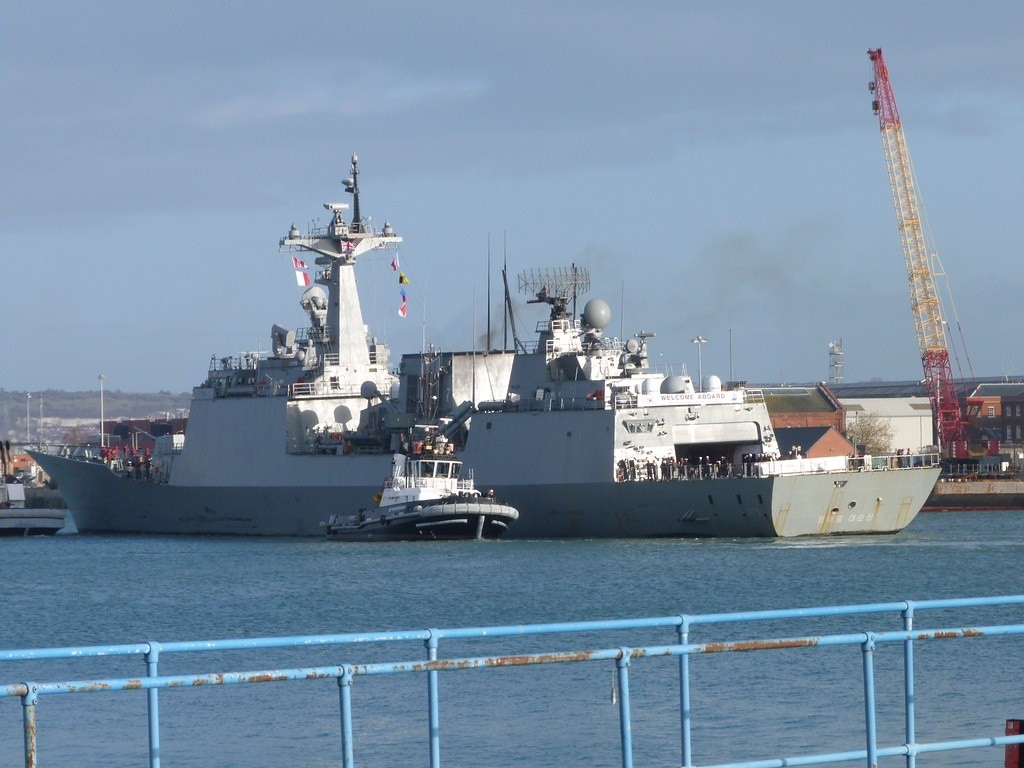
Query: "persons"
424 464 433 476
743 452 807 476
897 448 905 468
644 455 730 478
101 444 150 479
221 356 232 369
847 452 864 469
906 448 913 467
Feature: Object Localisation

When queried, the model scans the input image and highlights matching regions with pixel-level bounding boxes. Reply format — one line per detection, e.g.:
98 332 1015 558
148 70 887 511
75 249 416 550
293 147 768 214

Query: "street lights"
24 392 32 444
691 336 709 393
97 374 105 446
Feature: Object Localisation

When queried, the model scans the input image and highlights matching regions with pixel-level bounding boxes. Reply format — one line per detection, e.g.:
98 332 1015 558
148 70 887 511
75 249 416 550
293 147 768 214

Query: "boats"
317 307 521 540
0 475 66 538
24 154 946 537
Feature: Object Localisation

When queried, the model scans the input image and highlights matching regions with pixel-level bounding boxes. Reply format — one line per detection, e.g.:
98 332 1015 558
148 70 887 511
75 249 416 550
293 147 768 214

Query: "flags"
400 288 408 302
391 254 399 271
398 303 407 318
296 271 310 286
399 272 409 284
292 257 309 269
341 240 354 252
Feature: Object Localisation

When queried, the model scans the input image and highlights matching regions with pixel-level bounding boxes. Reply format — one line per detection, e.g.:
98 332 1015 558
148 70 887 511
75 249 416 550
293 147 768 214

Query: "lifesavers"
464 492 471 498
472 492 480 498
326 525 332 534
380 515 385 524
